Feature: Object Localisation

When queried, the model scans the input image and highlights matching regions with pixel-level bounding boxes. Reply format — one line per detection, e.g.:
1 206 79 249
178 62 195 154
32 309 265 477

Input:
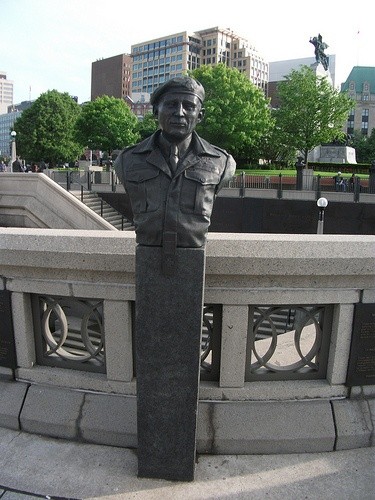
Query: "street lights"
11 131 17 161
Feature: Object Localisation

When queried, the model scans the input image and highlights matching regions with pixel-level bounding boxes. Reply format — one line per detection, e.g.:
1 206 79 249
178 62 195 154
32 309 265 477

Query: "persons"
112 74 236 249
12 156 48 174
332 171 346 192
75 160 78 170
349 173 360 192
0 160 7 173
